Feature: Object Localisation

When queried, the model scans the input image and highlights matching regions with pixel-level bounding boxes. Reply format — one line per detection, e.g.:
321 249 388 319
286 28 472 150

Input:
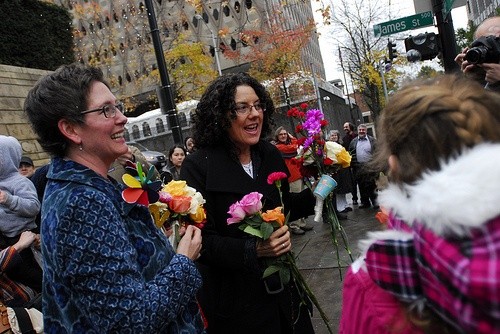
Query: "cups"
313 174 338 200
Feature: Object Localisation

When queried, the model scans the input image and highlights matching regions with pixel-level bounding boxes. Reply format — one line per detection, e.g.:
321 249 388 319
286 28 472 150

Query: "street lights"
310 62 325 120
195 14 222 76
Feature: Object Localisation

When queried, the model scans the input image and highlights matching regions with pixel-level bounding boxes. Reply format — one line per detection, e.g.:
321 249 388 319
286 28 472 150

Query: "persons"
107 136 195 190
341 122 361 206
338 73 500 334
454 15 500 93
318 128 328 143
326 130 351 219
0 134 50 334
22 63 207 334
350 124 381 209
183 73 316 334
268 122 317 235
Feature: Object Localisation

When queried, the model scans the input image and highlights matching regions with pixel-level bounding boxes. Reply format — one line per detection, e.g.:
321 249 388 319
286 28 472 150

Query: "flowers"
159 179 207 245
227 103 356 334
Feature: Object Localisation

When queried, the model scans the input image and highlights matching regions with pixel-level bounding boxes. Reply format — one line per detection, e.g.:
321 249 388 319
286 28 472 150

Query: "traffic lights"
384 56 392 64
387 41 398 61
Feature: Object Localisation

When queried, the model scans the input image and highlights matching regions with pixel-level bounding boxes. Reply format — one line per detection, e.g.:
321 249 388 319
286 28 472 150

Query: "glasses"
234 103 266 115
80 102 125 118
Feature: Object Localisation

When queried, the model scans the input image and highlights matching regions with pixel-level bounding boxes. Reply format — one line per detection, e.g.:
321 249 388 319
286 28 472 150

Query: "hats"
20 157 33 165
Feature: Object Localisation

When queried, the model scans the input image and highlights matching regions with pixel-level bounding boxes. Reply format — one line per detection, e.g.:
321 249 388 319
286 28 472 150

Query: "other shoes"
292 228 305 235
374 205 379 209
300 223 313 230
337 213 348 220
359 204 371 208
341 207 352 213
353 199 358 205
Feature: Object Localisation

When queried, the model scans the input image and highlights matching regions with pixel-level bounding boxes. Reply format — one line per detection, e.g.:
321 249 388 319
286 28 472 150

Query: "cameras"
466 35 500 66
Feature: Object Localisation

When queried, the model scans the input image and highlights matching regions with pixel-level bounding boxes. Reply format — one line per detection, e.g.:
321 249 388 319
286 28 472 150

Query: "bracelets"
132 153 136 164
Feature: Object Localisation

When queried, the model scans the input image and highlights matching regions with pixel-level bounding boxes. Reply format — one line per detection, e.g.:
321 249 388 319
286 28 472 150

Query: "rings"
281 243 286 248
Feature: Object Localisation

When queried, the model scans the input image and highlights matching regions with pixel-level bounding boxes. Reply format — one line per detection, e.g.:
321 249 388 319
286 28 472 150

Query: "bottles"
31 245 45 270
